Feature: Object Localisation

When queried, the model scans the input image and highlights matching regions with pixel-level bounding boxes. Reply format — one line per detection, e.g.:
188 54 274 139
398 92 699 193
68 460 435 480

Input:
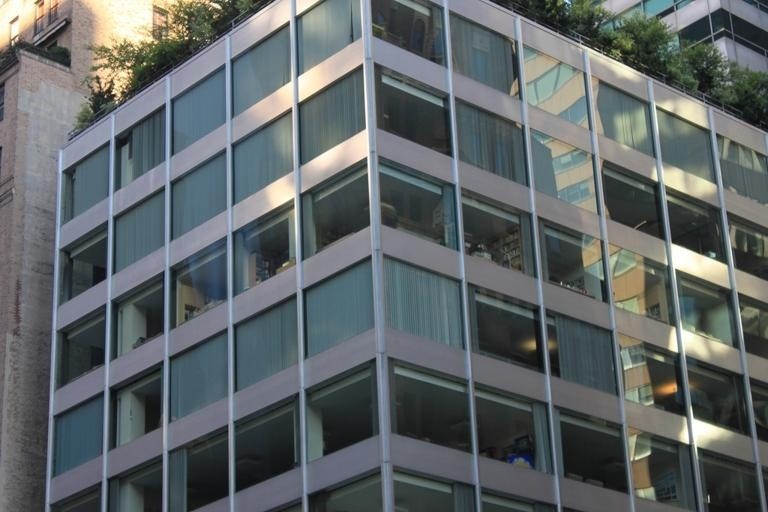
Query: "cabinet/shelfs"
649 462 685 508
488 225 527 272
